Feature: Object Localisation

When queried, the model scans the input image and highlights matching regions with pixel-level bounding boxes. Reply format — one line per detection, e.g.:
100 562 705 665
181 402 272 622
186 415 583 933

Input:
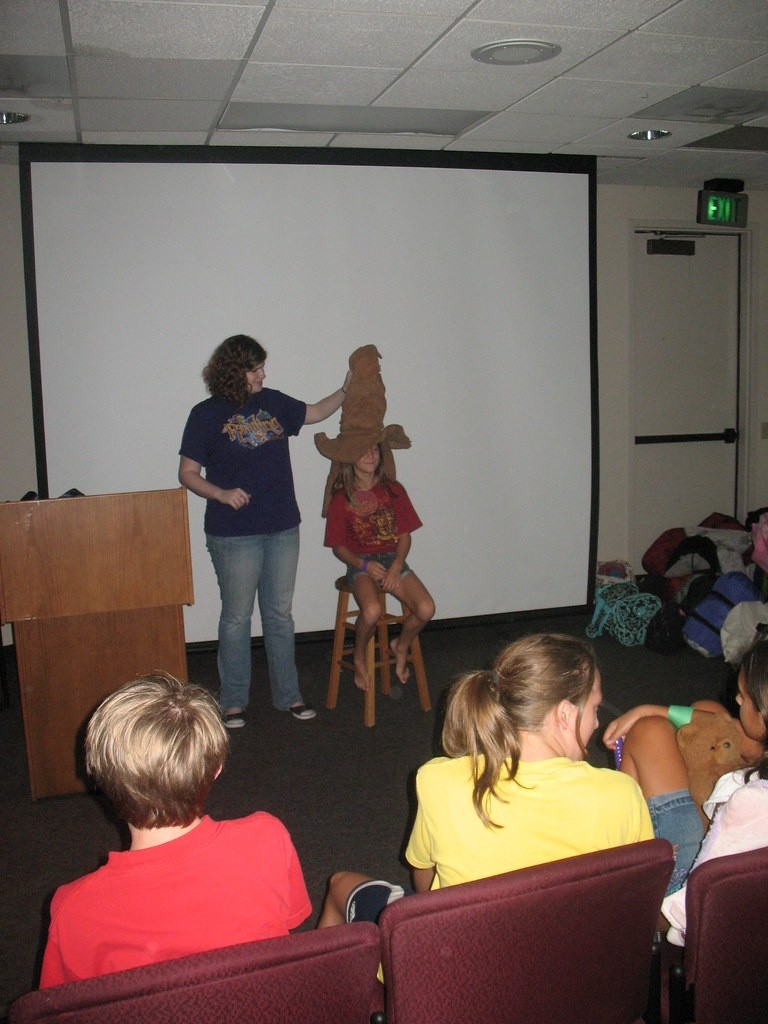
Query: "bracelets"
361 560 368 571
667 704 694 727
342 387 346 393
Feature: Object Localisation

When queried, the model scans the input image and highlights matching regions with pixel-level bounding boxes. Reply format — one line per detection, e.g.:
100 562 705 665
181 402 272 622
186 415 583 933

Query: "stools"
327 575 431 727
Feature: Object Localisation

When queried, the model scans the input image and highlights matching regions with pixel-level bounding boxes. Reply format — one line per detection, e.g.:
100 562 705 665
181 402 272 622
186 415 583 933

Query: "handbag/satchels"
586 581 662 647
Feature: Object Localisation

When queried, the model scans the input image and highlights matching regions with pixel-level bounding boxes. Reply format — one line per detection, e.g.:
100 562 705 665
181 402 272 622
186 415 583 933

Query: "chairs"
9 835 768 1024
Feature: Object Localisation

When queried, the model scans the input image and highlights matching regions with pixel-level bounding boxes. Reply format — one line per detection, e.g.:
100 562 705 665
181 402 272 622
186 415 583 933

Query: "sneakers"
289 703 317 720
221 712 246 728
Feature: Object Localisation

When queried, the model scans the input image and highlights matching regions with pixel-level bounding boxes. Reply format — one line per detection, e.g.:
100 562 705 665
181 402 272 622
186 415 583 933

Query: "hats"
313 344 412 465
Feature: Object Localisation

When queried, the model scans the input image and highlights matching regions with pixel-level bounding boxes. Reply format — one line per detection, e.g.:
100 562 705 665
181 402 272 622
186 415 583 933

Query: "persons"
324 444 435 691
602 642 768 952
179 335 353 728
315 632 654 983
40 674 313 986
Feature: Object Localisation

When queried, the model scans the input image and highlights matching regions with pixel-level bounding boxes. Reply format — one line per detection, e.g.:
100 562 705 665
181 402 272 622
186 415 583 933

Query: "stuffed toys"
676 711 744 833
